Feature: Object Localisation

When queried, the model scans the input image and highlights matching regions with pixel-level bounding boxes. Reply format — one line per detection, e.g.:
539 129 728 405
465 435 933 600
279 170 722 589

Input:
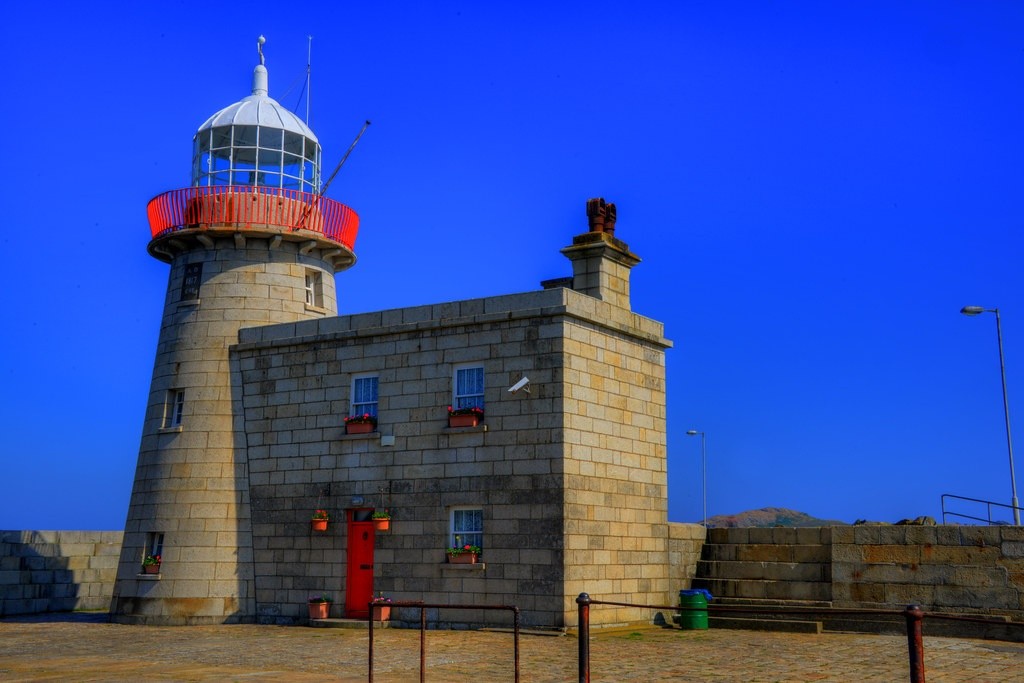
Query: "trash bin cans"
679 588 710 630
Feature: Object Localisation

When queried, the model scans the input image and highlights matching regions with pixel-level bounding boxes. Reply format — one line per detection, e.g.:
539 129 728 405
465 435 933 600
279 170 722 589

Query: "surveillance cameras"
508 376 529 394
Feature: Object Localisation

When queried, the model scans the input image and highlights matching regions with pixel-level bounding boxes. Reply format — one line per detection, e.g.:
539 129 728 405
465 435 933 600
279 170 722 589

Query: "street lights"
958 306 1021 525
685 429 707 528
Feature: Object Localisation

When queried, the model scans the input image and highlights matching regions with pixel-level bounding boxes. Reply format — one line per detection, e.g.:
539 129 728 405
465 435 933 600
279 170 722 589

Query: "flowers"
371 591 392 603
142 555 162 565
307 593 331 602
445 536 482 559
344 414 377 423
447 404 485 416
373 509 391 518
311 508 333 520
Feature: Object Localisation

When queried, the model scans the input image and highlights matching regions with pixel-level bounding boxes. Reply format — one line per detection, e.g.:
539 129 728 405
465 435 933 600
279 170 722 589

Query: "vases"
345 423 375 433
145 565 159 573
313 519 328 530
448 415 479 426
448 553 478 564
373 607 391 621
373 519 390 531
308 603 334 619
